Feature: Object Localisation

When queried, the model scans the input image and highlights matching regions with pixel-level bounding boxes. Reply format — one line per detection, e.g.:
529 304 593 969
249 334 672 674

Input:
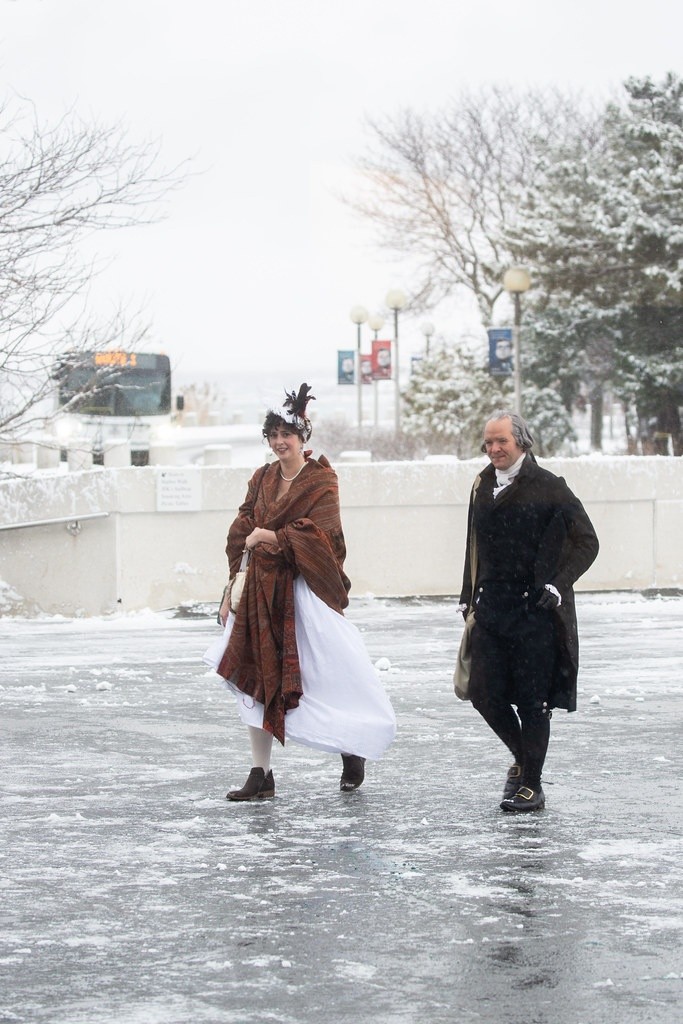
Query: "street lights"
368 311 387 435
420 320 438 361
385 285 417 446
506 263 532 422
349 303 372 450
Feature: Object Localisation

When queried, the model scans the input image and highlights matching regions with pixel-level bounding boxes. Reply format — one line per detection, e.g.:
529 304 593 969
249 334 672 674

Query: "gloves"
536 590 559 612
462 608 470 621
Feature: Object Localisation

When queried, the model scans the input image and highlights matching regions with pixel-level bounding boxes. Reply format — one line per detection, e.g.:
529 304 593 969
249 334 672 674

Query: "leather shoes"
500 762 545 812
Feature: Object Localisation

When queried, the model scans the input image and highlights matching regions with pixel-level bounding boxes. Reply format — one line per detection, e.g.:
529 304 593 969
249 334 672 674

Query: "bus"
48 347 185 466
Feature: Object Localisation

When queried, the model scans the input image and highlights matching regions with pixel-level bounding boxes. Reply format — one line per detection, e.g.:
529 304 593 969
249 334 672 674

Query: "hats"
267 383 316 443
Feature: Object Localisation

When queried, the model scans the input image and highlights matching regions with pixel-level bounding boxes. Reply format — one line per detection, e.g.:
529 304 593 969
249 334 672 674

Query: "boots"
340 753 366 791
226 767 275 800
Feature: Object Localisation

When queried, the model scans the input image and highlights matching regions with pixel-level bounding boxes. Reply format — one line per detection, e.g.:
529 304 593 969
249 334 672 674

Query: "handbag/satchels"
227 566 250 616
453 611 476 700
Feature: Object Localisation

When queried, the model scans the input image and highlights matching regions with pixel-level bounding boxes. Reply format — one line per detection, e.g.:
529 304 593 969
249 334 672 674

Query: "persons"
217 382 366 802
458 408 599 812
